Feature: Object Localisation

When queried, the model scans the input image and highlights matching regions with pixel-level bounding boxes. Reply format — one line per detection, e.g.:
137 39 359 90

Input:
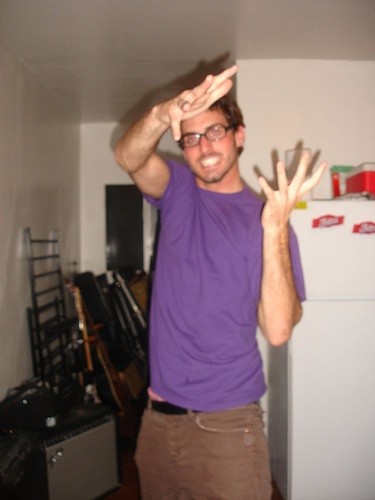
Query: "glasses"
178 123 234 150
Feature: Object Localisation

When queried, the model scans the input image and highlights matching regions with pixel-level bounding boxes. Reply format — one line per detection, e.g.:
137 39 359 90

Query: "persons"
115 64 327 499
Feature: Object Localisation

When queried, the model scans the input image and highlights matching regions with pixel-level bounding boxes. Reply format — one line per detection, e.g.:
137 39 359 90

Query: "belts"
148 399 200 415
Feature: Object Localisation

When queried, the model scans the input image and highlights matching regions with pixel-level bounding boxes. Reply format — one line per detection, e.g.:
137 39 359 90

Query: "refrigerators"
267 200 374 499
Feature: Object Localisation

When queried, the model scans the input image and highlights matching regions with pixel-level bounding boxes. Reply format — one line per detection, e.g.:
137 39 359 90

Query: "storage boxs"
346 162 375 193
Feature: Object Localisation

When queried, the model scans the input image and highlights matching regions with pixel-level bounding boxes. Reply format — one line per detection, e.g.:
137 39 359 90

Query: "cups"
286 148 313 199
